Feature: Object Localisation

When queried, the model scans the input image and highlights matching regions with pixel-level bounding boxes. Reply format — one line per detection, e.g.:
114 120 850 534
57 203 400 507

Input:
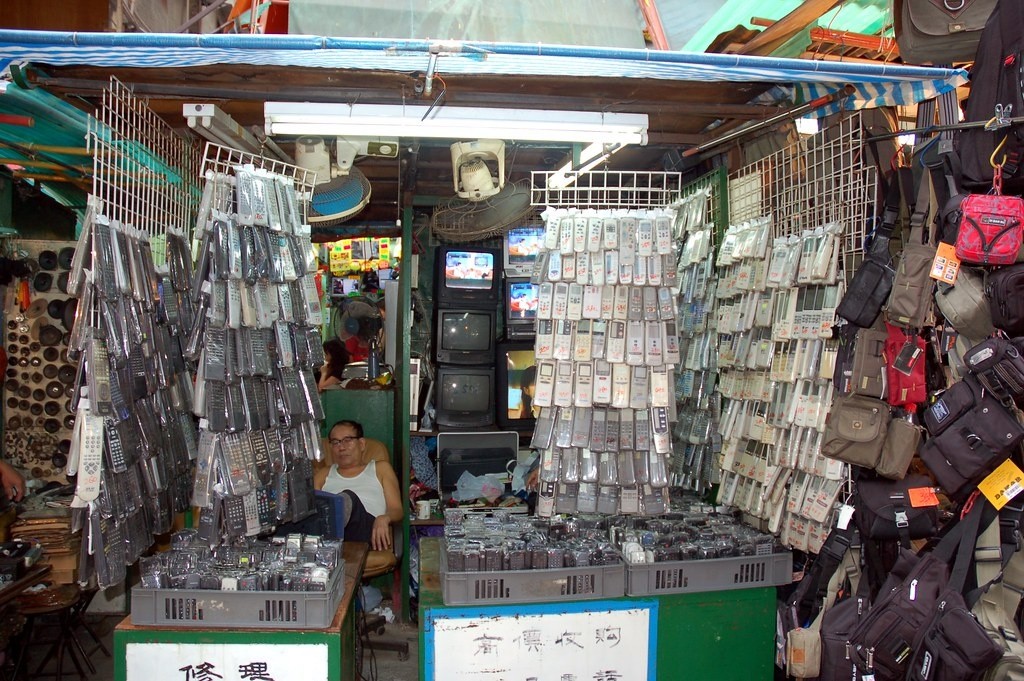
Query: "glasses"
328 436 360 448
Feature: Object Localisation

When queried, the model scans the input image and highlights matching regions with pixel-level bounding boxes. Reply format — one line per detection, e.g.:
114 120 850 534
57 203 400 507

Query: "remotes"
63 167 342 600
444 213 845 600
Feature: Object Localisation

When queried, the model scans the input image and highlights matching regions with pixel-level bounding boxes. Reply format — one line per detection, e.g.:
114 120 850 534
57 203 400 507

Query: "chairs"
360 525 410 661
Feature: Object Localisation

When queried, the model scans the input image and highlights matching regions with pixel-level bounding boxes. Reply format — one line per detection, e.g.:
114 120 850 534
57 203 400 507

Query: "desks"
112 542 368 681
0 564 53 608
9 583 88 681
321 383 394 466
417 536 777 681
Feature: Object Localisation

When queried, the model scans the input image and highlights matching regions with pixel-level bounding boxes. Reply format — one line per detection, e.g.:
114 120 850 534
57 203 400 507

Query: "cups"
415 501 430 520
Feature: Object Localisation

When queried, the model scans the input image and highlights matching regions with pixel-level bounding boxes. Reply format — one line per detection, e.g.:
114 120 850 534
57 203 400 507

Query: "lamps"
547 142 628 190
183 103 278 171
263 101 649 146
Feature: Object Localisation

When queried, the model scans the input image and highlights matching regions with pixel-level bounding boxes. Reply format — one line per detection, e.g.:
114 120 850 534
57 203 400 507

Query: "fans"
292 136 372 228
333 295 384 379
431 139 541 242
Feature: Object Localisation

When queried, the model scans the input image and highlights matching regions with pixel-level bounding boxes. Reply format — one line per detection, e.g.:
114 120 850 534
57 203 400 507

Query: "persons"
335 281 343 294
516 366 542 419
318 338 350 392
454 262 489 279
526 466 539 490
0 459 26 502
517 238 529 256
314 421 404 551
518 294 529 311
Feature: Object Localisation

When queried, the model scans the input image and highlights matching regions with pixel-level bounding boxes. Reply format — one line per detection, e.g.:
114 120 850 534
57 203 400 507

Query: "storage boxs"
38 551 80 584
130 558 346 630
608 541 794 597
436 537 625 606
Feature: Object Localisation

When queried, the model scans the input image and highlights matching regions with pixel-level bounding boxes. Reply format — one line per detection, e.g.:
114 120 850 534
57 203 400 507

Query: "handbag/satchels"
777 0 1024 680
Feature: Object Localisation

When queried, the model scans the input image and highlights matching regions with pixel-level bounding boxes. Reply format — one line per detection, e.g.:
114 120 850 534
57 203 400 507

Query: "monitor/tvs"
436 309 497 367
429 244 501 311
435 366 496 428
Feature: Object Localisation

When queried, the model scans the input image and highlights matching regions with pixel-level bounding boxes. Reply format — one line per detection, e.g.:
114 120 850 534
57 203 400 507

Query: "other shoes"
336 492 353 527
